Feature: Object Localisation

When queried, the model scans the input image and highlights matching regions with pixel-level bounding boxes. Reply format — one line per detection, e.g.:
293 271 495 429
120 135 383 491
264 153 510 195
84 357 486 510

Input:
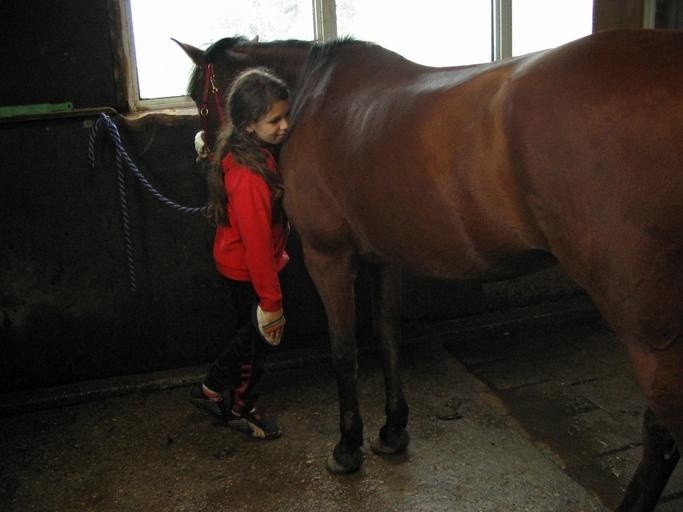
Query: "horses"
169 28 683 512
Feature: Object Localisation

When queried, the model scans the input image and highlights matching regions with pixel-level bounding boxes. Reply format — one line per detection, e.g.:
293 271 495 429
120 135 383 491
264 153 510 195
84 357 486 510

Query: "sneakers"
226 406 278 439
189 381 226 418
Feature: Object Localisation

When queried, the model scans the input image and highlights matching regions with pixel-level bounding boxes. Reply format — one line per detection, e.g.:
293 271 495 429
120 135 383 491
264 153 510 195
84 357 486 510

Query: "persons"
184 67 296 441
